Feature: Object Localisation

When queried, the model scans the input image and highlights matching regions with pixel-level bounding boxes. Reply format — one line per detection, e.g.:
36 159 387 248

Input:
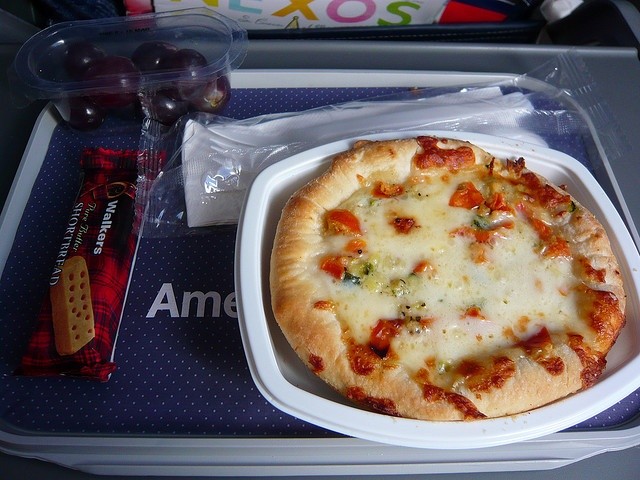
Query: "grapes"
61 43 228 129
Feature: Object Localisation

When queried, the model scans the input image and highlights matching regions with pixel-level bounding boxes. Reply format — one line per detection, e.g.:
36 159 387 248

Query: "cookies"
52 255 98 355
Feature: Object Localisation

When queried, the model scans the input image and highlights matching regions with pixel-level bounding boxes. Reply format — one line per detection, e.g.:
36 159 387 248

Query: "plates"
234 129 640 448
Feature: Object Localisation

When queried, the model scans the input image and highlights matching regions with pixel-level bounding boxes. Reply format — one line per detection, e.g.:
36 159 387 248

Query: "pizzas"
270 137 627 423
